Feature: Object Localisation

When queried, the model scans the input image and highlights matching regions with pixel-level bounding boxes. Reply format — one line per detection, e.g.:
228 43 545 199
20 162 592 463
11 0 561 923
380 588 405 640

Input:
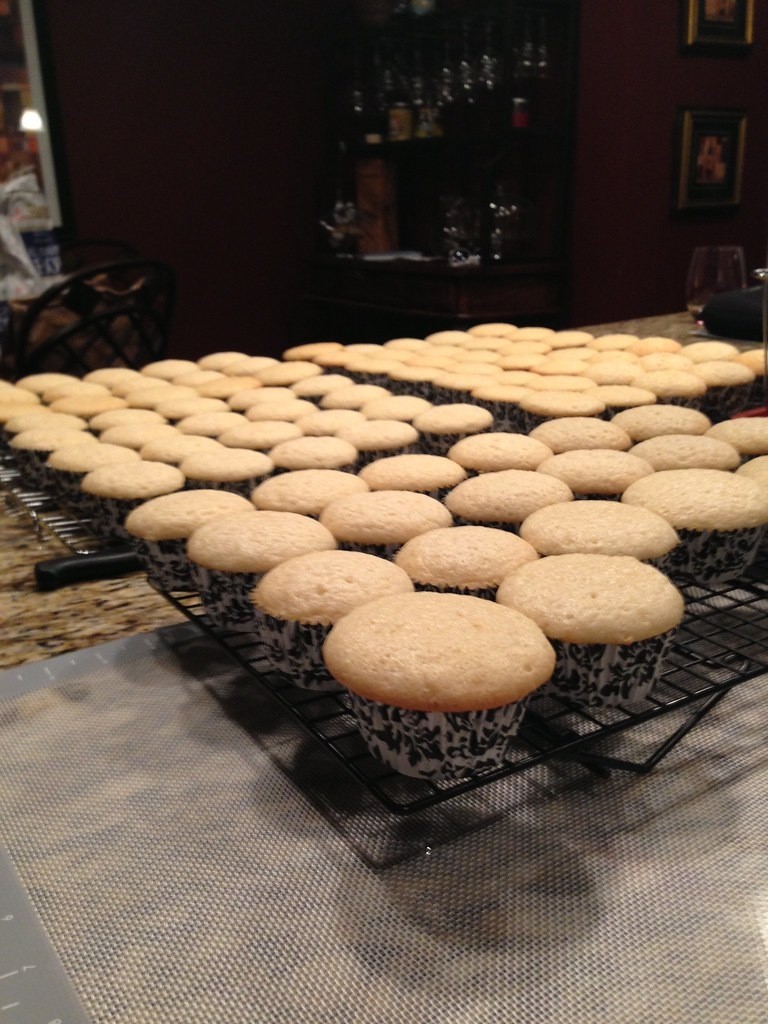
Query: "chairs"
14 238 176 380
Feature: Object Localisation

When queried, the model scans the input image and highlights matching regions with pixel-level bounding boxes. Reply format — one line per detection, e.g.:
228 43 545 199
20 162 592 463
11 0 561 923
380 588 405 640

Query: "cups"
686 245 747 318
437 197 482 267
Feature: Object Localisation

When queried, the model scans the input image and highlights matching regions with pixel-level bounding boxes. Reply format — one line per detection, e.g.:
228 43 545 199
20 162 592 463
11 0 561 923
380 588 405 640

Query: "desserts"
0 324 768 777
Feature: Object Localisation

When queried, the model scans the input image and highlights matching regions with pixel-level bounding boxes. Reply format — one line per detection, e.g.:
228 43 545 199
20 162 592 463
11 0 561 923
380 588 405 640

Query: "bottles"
352 40 546 141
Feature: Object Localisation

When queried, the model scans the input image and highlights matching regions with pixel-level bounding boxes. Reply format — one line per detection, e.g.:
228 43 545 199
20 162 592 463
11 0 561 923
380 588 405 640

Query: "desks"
0 315 768 1024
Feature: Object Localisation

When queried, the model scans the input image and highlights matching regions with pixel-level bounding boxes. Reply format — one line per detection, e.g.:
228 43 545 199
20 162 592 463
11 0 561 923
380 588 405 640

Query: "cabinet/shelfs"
299 0 582 340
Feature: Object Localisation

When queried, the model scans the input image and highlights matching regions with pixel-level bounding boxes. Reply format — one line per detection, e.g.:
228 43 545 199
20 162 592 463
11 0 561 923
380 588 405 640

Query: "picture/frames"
679 0 756 55
0 0 75 247
668 103 748 217
